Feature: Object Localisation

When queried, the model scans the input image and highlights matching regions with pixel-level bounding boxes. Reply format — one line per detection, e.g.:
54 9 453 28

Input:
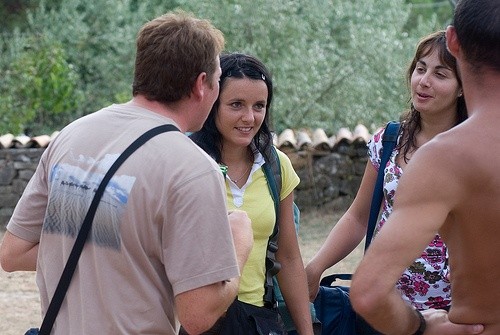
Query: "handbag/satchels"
313 274 383 335
217 300 282 335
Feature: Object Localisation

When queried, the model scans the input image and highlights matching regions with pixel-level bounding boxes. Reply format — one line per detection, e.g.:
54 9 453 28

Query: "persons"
349 0 500 334
305 29 453 313
0 12 254 334
185 52 315 335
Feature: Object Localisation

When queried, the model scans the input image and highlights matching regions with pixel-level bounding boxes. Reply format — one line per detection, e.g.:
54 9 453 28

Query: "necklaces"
228 162 252 183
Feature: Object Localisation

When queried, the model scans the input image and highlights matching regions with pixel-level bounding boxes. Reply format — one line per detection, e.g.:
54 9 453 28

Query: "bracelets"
414 310 426 335
